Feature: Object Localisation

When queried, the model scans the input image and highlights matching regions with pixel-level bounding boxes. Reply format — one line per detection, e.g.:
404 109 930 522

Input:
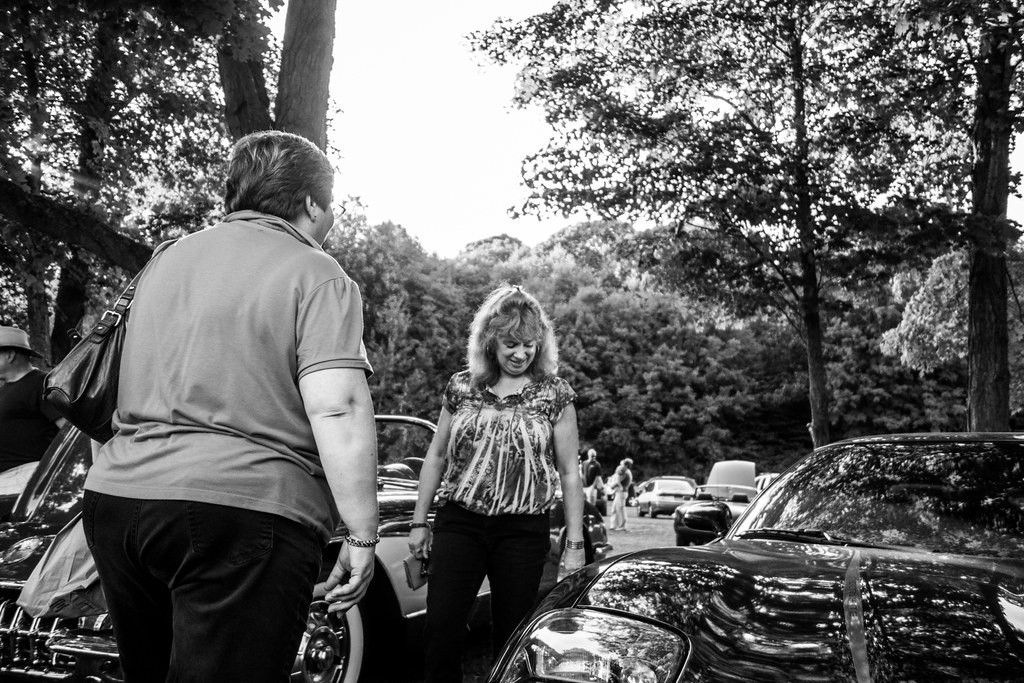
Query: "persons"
407 286 587 683
81 134 380 682
380 419 407 464
581 449 634 531
0 325 64 475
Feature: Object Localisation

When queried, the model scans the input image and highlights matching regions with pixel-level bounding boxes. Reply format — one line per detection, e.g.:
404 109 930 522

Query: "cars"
2 413 617 683
754 472 780 494
626 476 698 508
486 433 1023 683
635 479 696 519
673 459 761 547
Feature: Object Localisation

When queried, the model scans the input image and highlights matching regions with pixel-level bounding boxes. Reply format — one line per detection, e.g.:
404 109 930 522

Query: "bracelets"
344 528 380 547
565 538 584 550
408 522 430 528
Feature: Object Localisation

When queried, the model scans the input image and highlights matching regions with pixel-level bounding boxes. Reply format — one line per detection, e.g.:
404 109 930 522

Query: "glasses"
330 201 346 219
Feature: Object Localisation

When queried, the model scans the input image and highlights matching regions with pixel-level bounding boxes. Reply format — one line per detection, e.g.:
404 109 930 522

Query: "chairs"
887 484 965 526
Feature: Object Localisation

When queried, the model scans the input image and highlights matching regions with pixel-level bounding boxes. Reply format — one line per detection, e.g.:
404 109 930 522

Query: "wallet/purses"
403 544 431 591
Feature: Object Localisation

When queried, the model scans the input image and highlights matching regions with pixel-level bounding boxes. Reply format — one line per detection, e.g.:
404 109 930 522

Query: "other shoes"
615 527 625 531
610 526 614 529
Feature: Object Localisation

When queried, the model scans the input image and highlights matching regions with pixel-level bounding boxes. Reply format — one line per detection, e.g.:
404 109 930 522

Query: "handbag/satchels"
41 240 180 445
